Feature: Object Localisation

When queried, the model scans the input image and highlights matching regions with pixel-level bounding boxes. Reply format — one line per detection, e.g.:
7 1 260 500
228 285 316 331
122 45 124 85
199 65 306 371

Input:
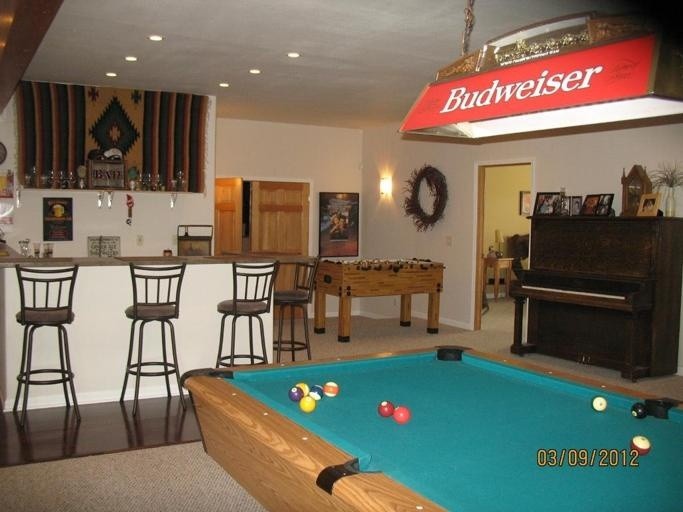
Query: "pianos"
507 216 682 381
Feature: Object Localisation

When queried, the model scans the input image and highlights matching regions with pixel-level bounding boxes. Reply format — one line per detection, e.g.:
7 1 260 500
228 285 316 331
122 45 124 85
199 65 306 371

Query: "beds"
507 233 528 279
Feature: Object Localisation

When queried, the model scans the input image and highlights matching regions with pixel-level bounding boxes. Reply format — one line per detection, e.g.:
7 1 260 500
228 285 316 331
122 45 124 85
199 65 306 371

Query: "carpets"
0 440 266 512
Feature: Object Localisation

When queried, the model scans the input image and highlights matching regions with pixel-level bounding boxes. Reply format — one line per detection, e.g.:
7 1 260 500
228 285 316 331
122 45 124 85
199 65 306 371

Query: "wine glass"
29 166 77 188
135 172 162 192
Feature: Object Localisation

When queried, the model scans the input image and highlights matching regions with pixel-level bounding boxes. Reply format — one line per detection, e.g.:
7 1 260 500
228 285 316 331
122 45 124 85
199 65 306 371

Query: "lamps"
399 1 683 141
493 228 504 259
378 175 393 197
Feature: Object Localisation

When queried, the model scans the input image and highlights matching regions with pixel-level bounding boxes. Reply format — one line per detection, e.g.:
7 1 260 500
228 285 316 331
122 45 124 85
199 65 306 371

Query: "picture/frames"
637 193 664 217
317 191 360 258
519 190 616 219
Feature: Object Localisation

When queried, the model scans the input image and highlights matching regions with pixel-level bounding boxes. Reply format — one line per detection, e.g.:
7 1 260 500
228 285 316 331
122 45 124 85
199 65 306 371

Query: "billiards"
379 400 394 415
631 401 646 418
391 406 410 424
290 380 339 413
592 395 606 411
632 436 650 456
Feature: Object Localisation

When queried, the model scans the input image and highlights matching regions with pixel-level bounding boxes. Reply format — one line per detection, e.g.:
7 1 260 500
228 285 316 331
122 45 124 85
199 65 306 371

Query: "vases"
665 188 675 218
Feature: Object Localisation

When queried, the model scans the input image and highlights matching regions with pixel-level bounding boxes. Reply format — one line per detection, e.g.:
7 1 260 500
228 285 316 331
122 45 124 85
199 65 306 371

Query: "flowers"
402 164 449 233
650 160 683 192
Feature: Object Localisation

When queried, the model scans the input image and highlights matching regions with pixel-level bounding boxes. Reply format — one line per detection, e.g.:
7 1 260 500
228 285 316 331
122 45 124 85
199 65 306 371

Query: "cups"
42 242 54 257
17 240 28 257
32 240 41 255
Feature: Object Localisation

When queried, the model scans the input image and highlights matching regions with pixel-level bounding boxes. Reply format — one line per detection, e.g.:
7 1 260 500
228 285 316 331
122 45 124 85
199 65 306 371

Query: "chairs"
211 258 280 367
120 261 187 416
12 263 80 425
269 255 316 361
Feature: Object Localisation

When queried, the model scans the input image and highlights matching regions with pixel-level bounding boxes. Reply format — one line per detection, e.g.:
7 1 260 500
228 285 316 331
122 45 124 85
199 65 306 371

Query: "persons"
330 215 349 235
330 212 339 235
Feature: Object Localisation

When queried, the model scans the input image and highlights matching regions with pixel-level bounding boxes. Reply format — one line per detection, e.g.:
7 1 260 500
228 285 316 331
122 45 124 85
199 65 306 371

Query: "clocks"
618 164 652 216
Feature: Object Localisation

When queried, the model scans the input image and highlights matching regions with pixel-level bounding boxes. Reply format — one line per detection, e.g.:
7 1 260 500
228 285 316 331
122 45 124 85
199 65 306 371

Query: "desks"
178 340 681 510
485 258 514 302
